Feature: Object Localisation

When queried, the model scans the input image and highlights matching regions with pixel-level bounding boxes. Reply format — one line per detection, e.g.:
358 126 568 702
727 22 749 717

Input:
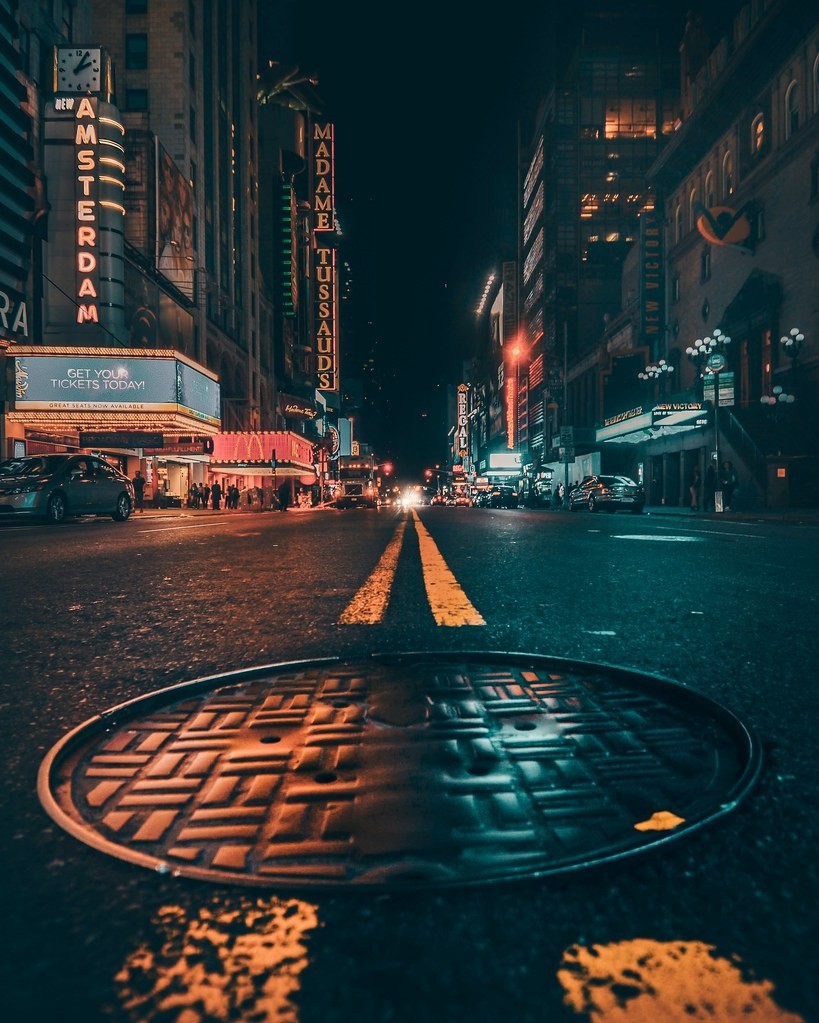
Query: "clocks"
53 45 106 97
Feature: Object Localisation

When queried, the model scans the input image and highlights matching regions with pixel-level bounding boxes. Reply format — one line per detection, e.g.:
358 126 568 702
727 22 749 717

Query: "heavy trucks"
334 453 381 509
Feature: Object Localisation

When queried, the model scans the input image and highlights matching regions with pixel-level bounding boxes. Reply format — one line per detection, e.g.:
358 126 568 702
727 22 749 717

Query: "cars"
568 474 646 514
0 453 136 526
381 471 562 510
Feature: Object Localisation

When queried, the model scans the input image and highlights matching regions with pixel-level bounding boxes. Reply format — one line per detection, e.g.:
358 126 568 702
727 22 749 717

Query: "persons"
528 488 535 510
132 471 146 513
690 459 737 511
192 480 246 510
554 481 579 507
278 478 292 512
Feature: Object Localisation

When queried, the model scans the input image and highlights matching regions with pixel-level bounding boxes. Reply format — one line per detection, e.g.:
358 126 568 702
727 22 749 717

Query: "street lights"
510 327 569 514
684 328 733 514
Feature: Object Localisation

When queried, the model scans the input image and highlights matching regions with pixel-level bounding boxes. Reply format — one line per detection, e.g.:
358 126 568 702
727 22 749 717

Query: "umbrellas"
526 465 555 478
